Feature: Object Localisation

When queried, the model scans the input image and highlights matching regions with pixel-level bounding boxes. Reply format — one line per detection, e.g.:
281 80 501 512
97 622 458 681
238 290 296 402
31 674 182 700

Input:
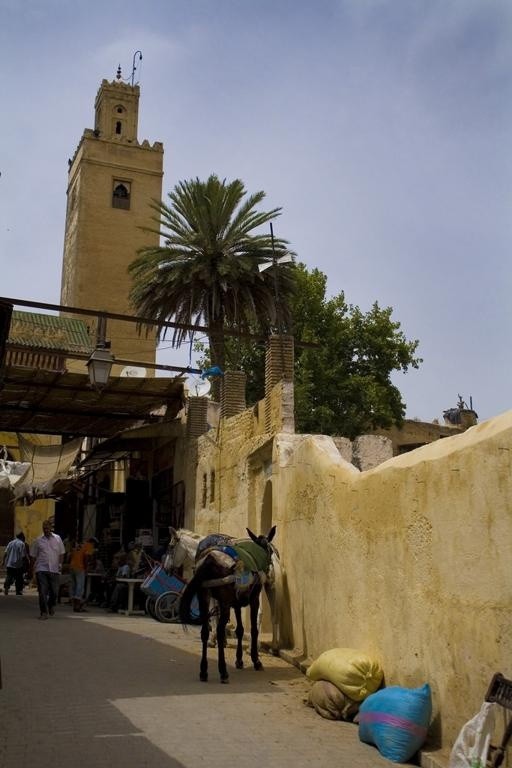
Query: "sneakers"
15 590 24 594
3 585 9 594
105 605 119 614
37 607 56 620
73 605 88 613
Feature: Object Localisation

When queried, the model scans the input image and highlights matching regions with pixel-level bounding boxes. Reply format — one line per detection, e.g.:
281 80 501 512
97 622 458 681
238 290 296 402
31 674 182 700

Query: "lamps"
85 316 116 391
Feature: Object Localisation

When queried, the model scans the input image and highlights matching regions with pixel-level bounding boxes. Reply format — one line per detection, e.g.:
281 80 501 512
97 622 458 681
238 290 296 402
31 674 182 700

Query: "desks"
115 577 147 616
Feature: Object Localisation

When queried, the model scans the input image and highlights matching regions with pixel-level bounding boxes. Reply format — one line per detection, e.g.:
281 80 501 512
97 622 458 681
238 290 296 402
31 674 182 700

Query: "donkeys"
179 523 278 682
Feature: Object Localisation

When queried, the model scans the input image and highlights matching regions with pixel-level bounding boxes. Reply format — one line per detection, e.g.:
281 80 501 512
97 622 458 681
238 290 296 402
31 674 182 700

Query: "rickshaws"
140 550 200 623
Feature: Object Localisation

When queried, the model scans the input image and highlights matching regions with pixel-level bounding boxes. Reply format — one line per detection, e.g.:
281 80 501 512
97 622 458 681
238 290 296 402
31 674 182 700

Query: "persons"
70 537 100 612
30 519 66 622
1 531 33 596
